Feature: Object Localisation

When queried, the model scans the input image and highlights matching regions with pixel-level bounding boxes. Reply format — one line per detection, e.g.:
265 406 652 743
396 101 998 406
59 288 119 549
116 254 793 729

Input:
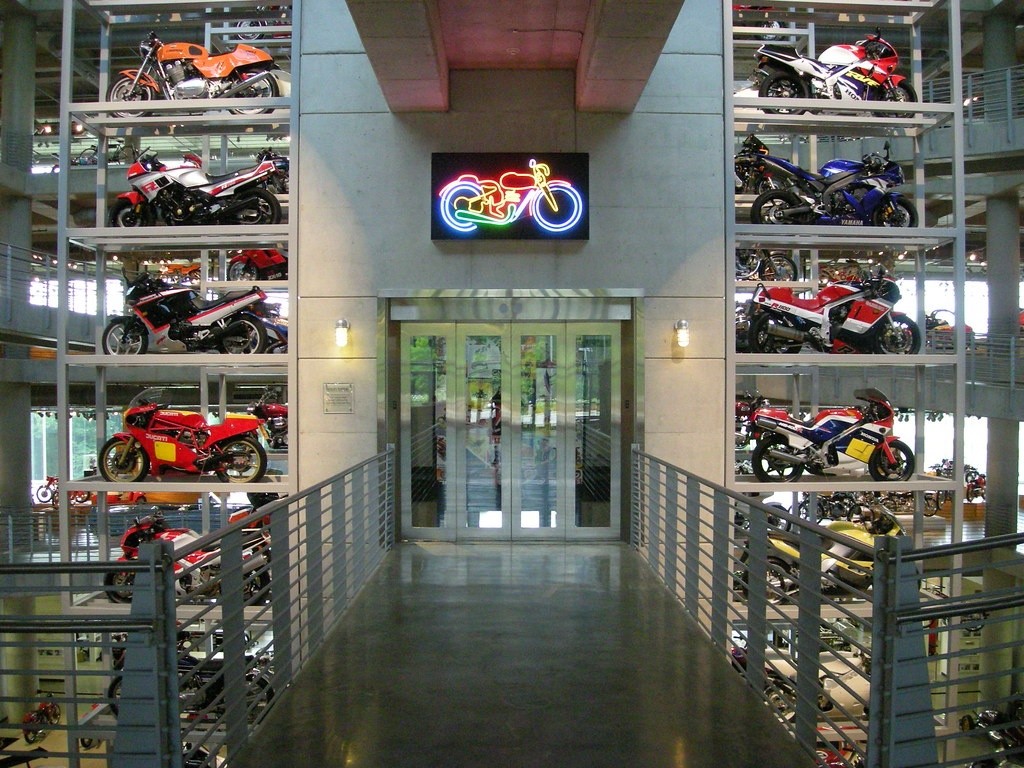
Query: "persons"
491 387 501 435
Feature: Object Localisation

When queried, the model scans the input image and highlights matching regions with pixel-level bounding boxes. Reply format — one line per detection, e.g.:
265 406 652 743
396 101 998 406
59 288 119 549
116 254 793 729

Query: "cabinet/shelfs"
58 0 297 768
724 0 965 768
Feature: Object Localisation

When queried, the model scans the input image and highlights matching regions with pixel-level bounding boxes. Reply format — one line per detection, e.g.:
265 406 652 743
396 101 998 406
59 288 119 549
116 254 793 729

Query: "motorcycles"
254 147 289 195
35 474 149 511
752 24 919 119
241 300 288 355
105 29 284 119
730 5 790 42
246 388 288 449
225 491 290 562
154 257 201 285
734 133 784 195
78 619 275 768
100 266 268 355
96 388 269 483
21 689 67 744
733 140 919 228
109 146 289 227
102 508 271 605
732 243 1024 768
226 247 290 282
